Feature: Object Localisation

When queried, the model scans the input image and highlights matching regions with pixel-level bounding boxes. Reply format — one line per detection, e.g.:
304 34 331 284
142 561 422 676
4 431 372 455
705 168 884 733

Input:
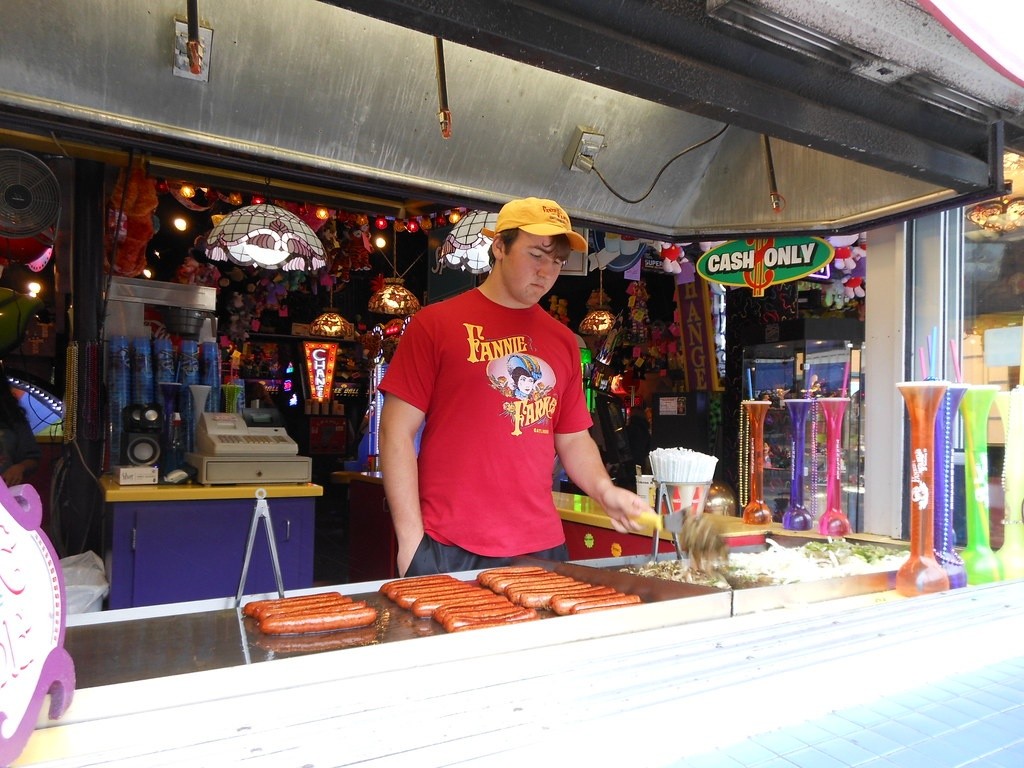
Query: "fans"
0 148 62 245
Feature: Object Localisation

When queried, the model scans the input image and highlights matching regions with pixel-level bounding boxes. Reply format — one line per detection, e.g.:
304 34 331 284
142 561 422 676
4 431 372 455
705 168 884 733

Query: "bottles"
166 412 182 468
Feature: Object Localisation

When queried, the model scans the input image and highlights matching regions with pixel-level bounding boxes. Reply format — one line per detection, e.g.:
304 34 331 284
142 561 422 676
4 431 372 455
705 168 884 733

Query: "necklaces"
812 399 817 516
1001 385 1024 525
740 401 749 507
924 376 963 565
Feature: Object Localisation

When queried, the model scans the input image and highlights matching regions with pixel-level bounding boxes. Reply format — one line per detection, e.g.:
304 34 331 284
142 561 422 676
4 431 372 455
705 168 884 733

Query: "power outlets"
172 20 214 84
562 126 605 174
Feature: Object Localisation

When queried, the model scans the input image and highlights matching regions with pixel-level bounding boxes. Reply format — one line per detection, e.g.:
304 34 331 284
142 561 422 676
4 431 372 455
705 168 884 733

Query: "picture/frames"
558 228 587 277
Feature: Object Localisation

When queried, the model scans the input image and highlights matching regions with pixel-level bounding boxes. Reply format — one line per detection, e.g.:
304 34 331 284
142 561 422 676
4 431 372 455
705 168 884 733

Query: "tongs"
235 489 285 607
649 484 684 563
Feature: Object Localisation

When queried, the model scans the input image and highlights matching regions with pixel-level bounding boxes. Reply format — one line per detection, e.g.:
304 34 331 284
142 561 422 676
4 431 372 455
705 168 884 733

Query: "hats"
481 197 589 255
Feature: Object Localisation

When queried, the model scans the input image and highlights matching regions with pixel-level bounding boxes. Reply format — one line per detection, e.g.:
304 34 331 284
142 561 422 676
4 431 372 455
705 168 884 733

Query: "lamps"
205 176 328 271
580 252 616 335
310 271 353 337
368 219 422 314
440 209 499 276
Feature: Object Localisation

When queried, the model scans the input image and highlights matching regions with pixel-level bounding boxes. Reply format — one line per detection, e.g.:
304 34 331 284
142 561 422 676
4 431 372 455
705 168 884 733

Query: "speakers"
140 405 162 432
121 404 144 432
118 430 168 480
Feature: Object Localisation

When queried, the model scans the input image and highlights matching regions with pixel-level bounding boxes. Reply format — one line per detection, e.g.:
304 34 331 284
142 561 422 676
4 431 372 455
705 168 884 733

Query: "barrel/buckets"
658 481 713 521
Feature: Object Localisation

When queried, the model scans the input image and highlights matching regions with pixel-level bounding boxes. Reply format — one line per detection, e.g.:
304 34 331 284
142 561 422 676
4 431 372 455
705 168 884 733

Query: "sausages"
477 566 644 615
242 592 377 635
378 575 542 633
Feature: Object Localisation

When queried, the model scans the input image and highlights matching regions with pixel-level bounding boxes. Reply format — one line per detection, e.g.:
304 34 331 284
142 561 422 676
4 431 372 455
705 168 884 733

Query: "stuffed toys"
821 230 868 311
104 168 373 341
601 229 695 273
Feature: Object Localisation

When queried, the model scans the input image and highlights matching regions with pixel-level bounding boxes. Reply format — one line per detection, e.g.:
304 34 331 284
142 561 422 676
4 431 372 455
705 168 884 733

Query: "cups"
304 397 344 417
109 337 241 470
635 474 654 498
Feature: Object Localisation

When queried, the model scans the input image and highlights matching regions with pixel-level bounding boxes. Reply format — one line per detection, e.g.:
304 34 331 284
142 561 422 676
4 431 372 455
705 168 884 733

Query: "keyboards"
218 435 288 444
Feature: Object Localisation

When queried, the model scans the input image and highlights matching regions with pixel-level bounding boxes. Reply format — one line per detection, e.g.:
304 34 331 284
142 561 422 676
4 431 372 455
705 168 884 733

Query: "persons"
0 366 41 489
376 197 658 578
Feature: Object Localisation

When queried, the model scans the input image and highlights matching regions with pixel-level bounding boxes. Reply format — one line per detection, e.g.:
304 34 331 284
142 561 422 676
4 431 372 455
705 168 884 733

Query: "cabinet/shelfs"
343 471 770 582
97 473 323 610
